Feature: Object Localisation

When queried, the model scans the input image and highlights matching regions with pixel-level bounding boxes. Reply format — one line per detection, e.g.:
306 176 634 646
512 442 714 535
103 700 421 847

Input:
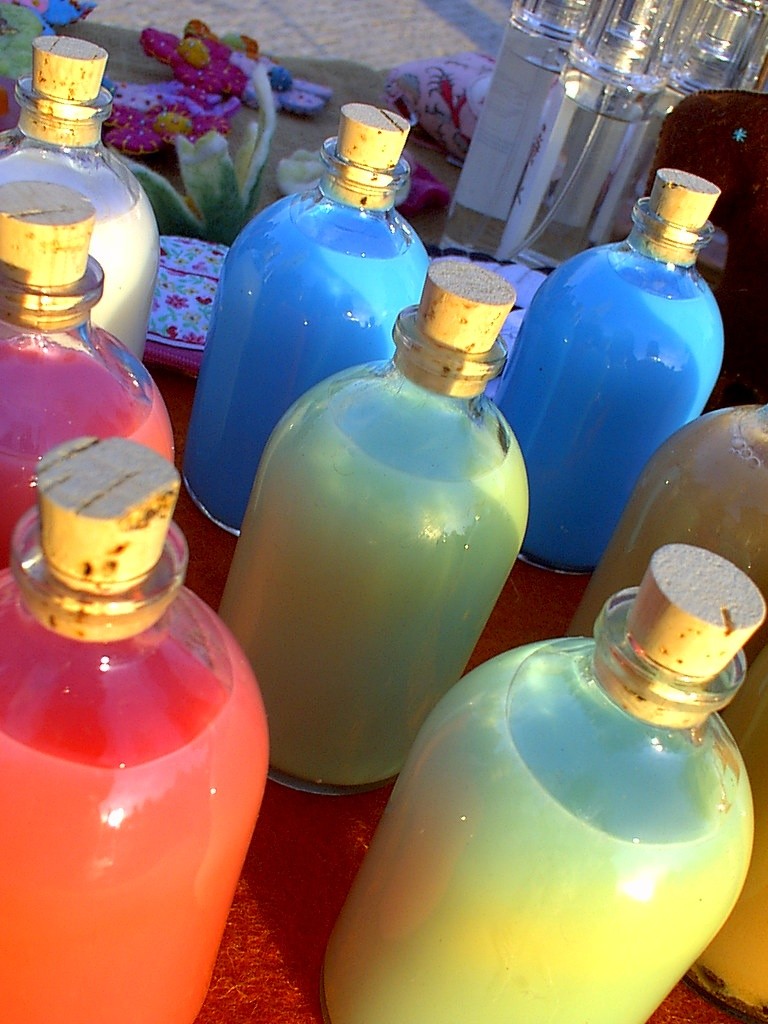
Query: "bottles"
1 35 161 362
322 543 765 1023
0 183 176 572
566 396 768 1019
185 101 431 541
1 435 268 1024
216 260 528 796
668 642 767 1023
487 171 728 573
